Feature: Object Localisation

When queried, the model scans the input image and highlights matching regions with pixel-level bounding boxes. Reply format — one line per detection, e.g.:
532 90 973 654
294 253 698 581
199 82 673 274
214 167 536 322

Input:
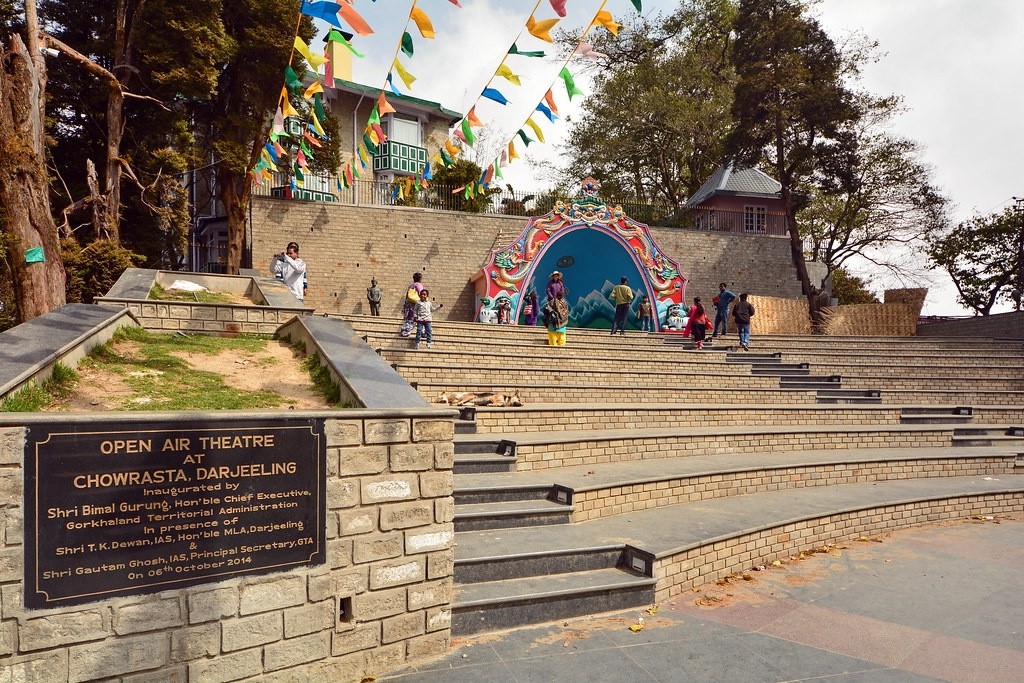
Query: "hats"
549 271 563 279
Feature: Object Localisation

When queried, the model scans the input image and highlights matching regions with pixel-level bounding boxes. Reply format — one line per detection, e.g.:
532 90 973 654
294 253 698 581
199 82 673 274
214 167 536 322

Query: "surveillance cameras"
46 48 60 57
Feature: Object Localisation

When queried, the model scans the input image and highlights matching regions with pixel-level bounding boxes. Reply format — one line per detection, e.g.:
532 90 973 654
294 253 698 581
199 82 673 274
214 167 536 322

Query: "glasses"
719 285 724 288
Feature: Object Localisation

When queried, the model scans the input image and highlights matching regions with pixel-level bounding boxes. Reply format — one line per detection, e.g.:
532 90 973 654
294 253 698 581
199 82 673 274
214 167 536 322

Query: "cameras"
277 255 284 261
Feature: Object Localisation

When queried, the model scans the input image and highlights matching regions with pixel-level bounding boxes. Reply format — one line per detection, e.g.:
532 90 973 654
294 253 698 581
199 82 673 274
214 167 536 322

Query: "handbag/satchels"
705 317 712 331
542 311 559 325
523 304 532 315
407 288 421 303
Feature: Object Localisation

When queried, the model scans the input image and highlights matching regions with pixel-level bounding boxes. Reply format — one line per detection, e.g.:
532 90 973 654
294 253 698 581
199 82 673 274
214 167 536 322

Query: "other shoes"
697 347 702 350
421 333 426 337
719 335 724 338
426 343 431 349
610 333 615 334
743 344 749 351
620 332 624 335
712 335 717 338
415 343 419 349
740 343 743 347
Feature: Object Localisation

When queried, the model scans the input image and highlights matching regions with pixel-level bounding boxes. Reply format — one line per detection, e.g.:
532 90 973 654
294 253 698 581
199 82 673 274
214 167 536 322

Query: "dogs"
434 389 524 408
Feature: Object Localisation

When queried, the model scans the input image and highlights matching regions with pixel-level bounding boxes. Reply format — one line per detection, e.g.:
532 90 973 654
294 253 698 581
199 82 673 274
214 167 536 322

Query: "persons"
611 276 633 336
270 246 306 302
542 291 568 345
401 272 427 337
413 289 443 349
546 271 564 301
523 292 540 326
637 295 653 331
712 283 736 337
499 303 510 324
732 293 755 351
366 277 382 316
275 242 307 295
689 297 706 350
